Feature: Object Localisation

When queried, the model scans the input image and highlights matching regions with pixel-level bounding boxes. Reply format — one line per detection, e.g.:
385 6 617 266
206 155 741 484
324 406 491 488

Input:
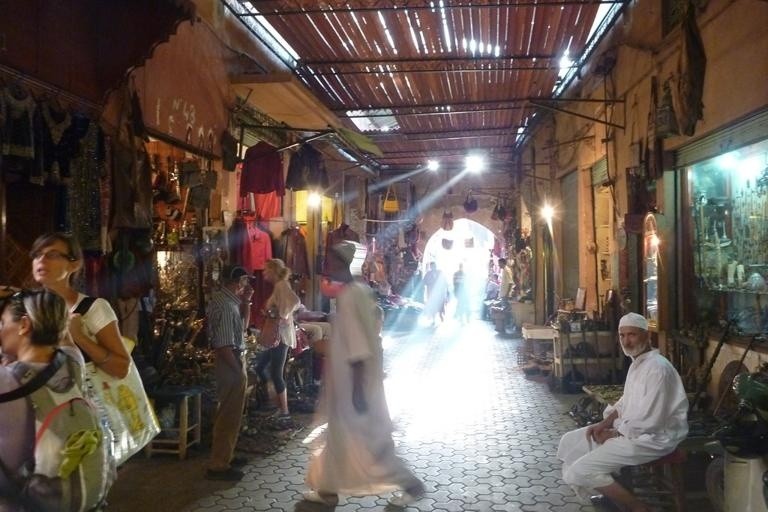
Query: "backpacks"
4 351 118 512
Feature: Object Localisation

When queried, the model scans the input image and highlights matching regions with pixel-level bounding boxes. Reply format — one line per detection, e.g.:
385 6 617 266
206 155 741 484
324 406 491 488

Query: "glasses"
36 251 70 260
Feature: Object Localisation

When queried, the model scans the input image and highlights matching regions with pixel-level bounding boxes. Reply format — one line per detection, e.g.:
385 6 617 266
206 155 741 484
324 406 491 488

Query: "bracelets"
242 301 252 306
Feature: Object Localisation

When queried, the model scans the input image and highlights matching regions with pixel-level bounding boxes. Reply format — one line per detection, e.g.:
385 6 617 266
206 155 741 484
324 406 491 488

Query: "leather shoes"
591 494 610 505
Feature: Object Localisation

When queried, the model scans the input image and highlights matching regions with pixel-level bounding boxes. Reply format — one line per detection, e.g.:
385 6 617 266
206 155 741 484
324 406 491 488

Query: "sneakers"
230 457 248 467
207 469 243 480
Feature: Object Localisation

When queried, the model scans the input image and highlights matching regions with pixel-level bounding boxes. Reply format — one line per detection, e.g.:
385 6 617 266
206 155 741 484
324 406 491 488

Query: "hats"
619 312 648 330
222 264 256 281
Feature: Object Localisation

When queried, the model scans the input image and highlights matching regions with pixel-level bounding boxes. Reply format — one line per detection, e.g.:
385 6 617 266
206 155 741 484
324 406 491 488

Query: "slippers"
302 491 331 504
388 490 421 506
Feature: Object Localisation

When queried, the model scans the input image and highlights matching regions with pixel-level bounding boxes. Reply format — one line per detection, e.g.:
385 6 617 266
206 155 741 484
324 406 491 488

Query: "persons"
0 286 86 512
255 259 301 429
422 258 516 326
29 233 131 378
556 311 690 512
302 244 424 508
208 266 254 481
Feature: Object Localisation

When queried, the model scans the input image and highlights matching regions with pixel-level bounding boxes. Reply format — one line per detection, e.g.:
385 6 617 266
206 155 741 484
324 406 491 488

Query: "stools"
144 387 202 462
619 452 681 511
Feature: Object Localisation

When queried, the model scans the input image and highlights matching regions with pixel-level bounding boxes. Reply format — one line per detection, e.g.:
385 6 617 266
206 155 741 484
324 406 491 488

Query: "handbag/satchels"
187 184 210 208
441 212 454 230
86 336 162 467
151 170 181 204
492 207 512 223
464 200 477 213
178 161 201 186
204 171 217 188
384 198 399 211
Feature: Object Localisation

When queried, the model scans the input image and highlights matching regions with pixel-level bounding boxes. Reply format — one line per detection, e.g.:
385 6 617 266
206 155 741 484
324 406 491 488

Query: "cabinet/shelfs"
551 329 616 392
